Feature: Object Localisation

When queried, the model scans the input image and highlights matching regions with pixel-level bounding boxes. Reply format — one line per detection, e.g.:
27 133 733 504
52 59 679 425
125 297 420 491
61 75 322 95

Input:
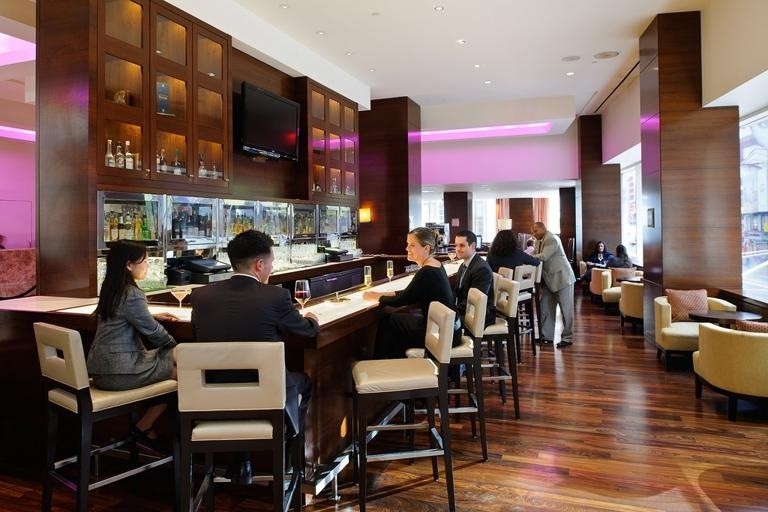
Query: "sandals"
131 422 166 452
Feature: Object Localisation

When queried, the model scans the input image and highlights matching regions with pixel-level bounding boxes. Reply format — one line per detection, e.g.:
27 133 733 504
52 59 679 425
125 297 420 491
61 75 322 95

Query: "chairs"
351 302 456 512
32 322 177 512
488 265 537 385
404 288 488 480
579 261 607 304
536 262 543 347
176 342 305 511
513 265 537 363
455 277 522 419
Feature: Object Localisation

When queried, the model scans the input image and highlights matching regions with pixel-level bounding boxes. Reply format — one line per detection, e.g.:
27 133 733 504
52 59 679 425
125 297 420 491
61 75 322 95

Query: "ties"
459 264 466 276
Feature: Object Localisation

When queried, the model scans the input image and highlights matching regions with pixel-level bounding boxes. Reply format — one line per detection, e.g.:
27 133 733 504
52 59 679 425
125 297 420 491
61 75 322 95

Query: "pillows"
611 266 636 287
664 289 708 322
735 320 768 332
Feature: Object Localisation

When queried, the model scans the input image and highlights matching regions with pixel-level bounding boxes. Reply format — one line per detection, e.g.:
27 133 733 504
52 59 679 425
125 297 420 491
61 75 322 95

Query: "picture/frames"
647 208 655 227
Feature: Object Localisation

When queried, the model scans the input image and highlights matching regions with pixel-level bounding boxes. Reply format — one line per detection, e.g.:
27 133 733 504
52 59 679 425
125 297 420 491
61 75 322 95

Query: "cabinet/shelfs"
35 1 232 296
338 206 351 238
219 199 254 245
290 203 316 241
294 76 360 208
97 191 165 257
166 195 217 244
257 201 287 245
317 205 336 238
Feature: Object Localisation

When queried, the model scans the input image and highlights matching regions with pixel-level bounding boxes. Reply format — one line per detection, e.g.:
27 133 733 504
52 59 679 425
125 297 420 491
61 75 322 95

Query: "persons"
193 229 320 488
454 228 496 338
84 239 177 455
608 243 632 270
531 225 576 349
524 237 534 253
576 241 613 284
374 227 463 357
485 230 539 274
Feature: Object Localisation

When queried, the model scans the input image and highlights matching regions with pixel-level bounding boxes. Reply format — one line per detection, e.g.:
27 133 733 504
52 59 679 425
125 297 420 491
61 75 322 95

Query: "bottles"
258 206 287 236
198 161 208 178
155 143 168 173
114 146 125 168
105 139 114 168
172 206 212 239
294 209 315 234
230 211 253 235
116 141 122 152
103 205 151 242
125 153 134 169
156 72 170 114
174 148 182 176
124 141 131 153
138 151 143 170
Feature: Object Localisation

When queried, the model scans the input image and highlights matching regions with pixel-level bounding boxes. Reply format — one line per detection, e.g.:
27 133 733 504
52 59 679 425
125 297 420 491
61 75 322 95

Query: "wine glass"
170 288 190 307
448 252 456 263
295 279 311 309
387 260 394 282
363 266 372 288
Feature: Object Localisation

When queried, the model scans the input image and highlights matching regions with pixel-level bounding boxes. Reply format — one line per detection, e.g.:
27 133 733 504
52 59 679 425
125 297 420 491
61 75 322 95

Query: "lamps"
498 219 512 231
358 208 371 223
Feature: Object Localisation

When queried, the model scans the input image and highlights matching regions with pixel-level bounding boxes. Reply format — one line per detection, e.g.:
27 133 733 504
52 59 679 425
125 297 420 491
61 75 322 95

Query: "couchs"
601 270 643 312
692 323 768 422
619 280 643 328
653 296 737 372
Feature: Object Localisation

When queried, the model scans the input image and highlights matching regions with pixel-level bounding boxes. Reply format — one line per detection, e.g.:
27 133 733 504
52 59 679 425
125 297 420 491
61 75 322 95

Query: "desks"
616 275 642 282
688 310 762 328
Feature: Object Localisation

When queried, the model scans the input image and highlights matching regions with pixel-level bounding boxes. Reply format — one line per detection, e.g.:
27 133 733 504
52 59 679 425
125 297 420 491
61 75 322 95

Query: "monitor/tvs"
190 258 230 273
238 82 299 162
477 236 482 250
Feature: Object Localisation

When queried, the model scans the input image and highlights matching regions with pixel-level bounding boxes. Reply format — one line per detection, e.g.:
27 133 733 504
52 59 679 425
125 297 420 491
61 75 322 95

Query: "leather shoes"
230 459 252 486
575 277 582 284
558 341 574 347
536 338 554 344
